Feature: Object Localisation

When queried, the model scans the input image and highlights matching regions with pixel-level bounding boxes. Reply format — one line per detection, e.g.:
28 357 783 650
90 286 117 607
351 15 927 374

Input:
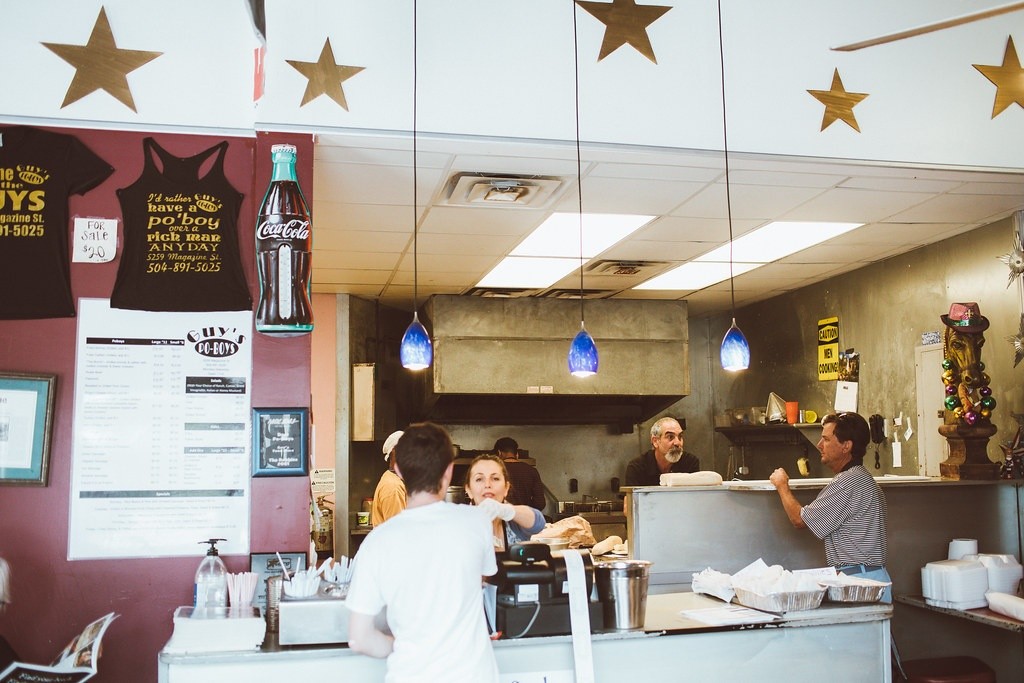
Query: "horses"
941 326 985 425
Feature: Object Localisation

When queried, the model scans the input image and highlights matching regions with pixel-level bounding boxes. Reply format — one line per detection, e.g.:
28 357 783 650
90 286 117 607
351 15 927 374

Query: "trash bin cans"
897 657 996 683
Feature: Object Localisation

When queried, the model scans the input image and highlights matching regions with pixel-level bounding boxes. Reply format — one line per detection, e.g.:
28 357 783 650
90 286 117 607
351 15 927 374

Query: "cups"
799 410 806 423
785 402 798 424
357 512 370 526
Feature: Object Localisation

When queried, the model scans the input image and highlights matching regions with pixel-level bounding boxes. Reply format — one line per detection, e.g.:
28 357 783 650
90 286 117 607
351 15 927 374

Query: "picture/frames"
0 370 56 488
252 408 308 477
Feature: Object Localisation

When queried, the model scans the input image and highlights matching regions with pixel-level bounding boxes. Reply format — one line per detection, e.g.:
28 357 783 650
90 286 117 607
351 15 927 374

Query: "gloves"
479 499 515 522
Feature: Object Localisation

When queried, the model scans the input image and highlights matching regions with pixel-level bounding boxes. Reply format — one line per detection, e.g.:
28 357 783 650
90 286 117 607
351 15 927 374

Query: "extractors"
409 293 691 435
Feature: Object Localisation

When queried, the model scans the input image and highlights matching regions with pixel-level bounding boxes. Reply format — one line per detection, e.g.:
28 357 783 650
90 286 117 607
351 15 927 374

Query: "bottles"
254 143 315 337
362 498 373 525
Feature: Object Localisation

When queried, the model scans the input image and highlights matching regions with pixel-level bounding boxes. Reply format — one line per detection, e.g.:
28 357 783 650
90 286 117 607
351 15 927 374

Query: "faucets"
582 494 598 511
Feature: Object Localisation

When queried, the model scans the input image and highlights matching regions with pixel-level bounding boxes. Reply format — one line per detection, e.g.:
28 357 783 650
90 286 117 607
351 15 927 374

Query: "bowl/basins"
282 570 322 598
320 576 351 597
921 554 1023 612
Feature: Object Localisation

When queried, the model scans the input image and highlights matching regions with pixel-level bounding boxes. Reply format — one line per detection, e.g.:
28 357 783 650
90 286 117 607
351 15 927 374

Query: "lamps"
717 0 751 371
400 1 432 371
568 0 599 376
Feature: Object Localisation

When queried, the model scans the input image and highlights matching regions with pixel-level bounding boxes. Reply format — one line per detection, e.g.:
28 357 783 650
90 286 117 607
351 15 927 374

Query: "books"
0 612 120 683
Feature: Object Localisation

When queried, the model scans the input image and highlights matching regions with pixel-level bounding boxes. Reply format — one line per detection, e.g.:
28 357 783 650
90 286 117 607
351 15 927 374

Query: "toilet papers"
948 536 979 561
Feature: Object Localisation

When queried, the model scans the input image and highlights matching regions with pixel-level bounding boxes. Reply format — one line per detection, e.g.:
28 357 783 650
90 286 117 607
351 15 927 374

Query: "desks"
894 594 1023 635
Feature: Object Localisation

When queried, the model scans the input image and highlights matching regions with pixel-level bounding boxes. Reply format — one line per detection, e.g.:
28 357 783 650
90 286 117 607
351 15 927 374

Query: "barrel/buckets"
446 486 465 504
593 560 654 630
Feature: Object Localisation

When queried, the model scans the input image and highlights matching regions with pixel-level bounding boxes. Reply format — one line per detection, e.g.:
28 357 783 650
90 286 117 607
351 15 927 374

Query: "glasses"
838 412 850 440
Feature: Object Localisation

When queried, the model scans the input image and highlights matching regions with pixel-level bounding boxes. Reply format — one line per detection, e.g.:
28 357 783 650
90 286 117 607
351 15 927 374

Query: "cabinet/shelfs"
352 362 398 441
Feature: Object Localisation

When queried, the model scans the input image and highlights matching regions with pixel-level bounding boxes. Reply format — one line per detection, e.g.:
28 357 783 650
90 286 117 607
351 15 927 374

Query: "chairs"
890 632 995 682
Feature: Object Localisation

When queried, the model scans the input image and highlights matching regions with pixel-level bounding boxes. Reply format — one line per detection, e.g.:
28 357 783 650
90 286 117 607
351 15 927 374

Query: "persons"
345 421 498 683
0 557 102 683
494 437 546 512
372 431 406 527
464 454 546 632
769 412 892 604
623 417 699 530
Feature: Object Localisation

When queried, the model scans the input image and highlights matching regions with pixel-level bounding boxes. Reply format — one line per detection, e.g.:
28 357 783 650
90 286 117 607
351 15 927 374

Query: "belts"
837 565 881 576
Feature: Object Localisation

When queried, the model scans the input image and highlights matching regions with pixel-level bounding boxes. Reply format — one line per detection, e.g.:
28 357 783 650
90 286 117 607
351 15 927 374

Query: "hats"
382 430 404 462
491 437 518 454
